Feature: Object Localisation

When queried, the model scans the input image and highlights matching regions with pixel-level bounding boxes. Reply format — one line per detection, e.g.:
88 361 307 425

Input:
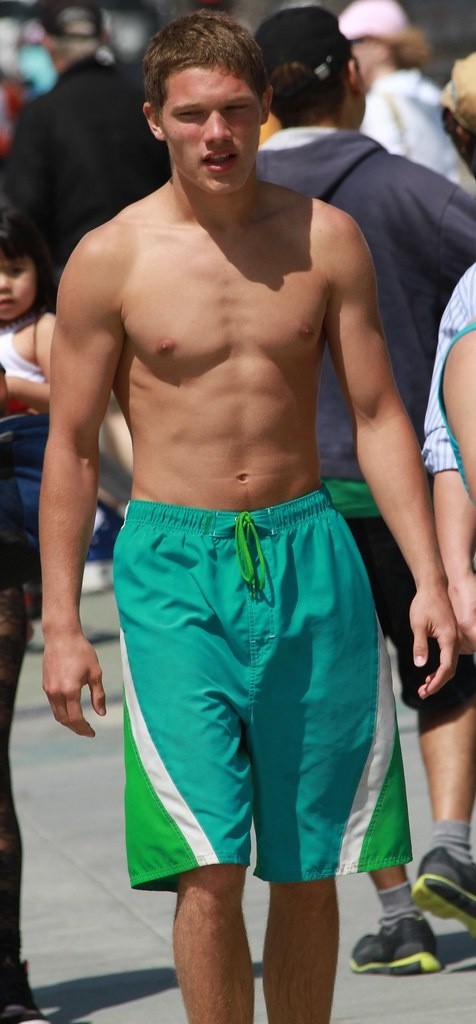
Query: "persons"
0 0 476 1024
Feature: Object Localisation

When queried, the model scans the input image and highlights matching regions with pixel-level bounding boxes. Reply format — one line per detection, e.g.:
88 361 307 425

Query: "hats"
253 6 364 106
40 0 103 40
337 0 409 49
438 52 476 134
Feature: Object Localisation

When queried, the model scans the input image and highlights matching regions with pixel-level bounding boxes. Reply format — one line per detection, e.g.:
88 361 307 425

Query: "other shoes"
81 558 113 597
0 960 51 1024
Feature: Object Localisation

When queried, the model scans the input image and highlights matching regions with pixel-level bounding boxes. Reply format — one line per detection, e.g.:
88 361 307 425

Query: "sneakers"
410 846 476 939
350 916 443 977
